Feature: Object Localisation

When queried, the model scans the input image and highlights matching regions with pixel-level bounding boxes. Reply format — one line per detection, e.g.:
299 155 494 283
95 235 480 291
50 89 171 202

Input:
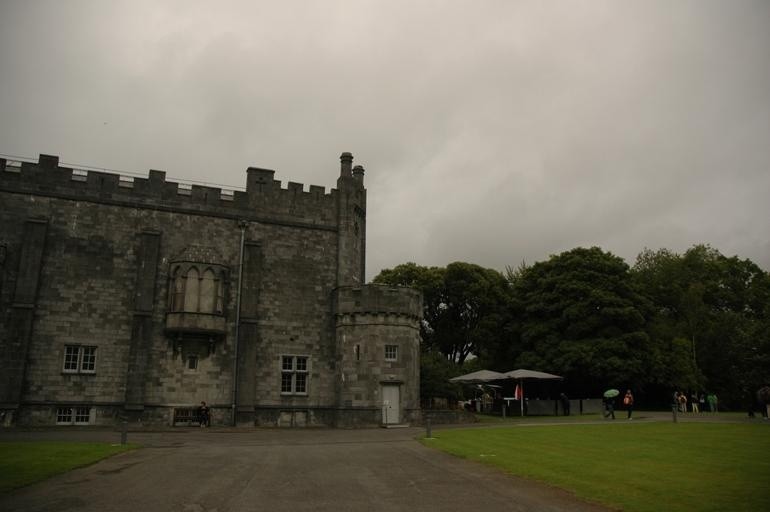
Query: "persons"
560 392 570 417
671 391 720 424
196 401 210 433
603 397 616 419
625 389 633 421
740 384 770 419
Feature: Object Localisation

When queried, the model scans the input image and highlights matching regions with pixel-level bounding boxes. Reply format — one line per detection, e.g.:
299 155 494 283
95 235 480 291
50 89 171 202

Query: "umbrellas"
603 389 619 397
448 369 508 416
504 368 564 417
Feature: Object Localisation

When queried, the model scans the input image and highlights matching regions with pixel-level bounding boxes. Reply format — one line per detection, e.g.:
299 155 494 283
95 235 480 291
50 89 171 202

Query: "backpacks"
680 395 685 403
623 397 630 404
